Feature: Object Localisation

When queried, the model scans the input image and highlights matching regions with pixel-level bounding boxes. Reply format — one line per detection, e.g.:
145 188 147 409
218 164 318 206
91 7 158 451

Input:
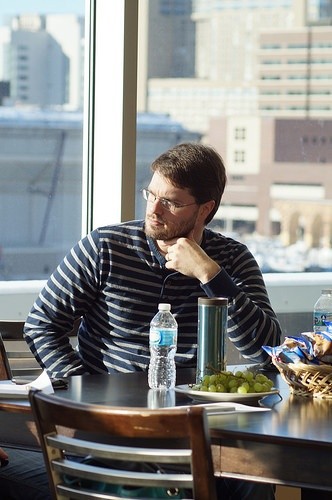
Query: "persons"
23 141 282 378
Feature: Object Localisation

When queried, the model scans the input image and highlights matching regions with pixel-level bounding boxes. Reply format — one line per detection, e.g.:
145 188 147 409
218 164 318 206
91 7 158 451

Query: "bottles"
312 290 332 340
148 302 178 390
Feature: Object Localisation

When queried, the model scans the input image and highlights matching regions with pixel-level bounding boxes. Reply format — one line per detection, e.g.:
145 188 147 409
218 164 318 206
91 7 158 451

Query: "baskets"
272 342 332 400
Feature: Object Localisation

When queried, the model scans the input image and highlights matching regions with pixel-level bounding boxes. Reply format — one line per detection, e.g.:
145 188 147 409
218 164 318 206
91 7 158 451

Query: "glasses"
140 187 197 211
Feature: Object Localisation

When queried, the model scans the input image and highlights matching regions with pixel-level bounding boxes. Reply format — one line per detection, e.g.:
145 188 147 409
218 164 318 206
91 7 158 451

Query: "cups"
198 298 228 382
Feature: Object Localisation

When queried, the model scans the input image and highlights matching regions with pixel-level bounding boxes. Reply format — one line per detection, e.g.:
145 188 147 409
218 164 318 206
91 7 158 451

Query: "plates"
176 384 279 402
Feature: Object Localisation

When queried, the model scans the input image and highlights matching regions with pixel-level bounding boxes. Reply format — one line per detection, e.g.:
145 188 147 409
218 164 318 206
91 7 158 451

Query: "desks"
0 363 332 490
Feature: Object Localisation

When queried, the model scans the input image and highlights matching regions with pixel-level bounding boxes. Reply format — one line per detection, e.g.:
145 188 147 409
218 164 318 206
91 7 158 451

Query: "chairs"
0 322 85 380
29 390 219 500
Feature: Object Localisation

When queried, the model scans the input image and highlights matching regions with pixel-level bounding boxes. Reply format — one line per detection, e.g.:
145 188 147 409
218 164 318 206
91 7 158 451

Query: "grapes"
193 371 275 394
